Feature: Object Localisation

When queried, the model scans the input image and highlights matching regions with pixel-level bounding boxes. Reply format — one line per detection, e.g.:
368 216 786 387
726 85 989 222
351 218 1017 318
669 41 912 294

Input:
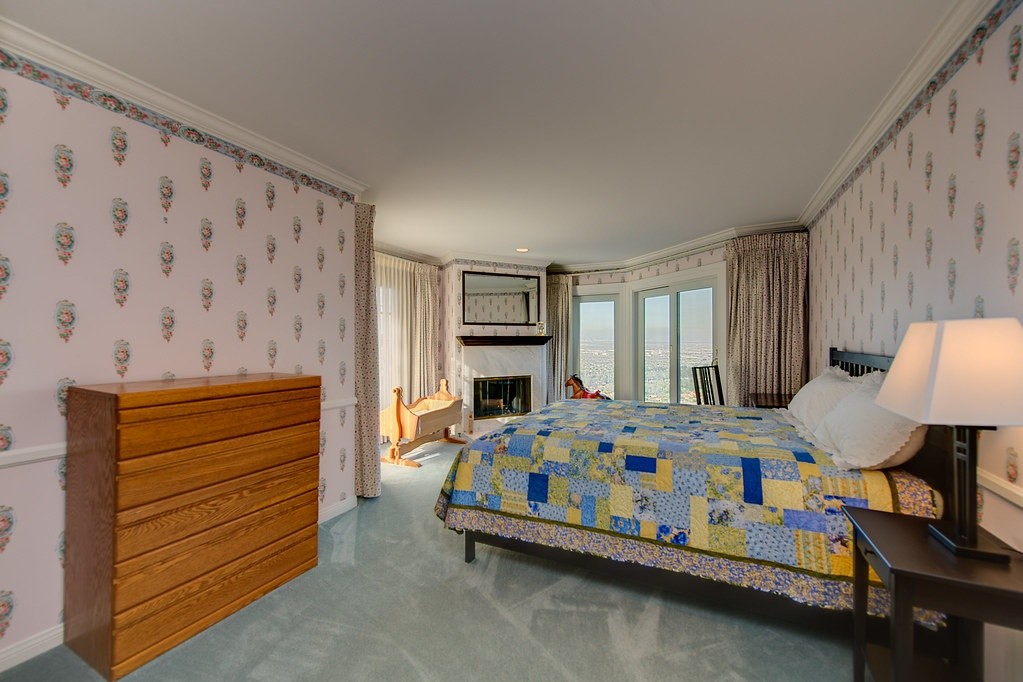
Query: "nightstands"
748 392 795 408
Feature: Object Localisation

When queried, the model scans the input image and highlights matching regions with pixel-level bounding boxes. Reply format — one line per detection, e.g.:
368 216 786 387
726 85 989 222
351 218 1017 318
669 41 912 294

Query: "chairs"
692 365 725 405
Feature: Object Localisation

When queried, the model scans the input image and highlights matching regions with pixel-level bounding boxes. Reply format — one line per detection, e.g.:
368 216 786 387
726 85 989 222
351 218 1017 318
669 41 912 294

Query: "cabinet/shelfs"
63 371 324 682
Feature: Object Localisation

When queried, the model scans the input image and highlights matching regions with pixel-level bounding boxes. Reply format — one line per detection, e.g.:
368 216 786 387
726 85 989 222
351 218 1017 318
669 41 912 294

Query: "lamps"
874 316 1023 563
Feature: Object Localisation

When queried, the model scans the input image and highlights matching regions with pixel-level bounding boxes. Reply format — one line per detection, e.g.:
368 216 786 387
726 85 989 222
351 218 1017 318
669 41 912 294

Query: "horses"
565 374 612 400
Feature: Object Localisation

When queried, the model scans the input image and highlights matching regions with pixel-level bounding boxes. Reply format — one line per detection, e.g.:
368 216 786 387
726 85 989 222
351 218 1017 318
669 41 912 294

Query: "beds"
434 347 975 645
379 379 468 467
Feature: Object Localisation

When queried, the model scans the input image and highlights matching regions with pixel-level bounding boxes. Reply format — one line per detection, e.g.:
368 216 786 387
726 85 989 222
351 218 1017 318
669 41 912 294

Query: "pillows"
772 364 929 474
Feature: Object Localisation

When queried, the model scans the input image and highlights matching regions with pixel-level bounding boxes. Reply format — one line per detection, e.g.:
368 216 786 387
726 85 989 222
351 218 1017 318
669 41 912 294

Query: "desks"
839 505 1023 682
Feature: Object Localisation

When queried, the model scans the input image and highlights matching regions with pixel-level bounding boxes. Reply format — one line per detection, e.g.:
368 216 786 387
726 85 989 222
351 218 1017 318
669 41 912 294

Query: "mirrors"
461 271 541 326
